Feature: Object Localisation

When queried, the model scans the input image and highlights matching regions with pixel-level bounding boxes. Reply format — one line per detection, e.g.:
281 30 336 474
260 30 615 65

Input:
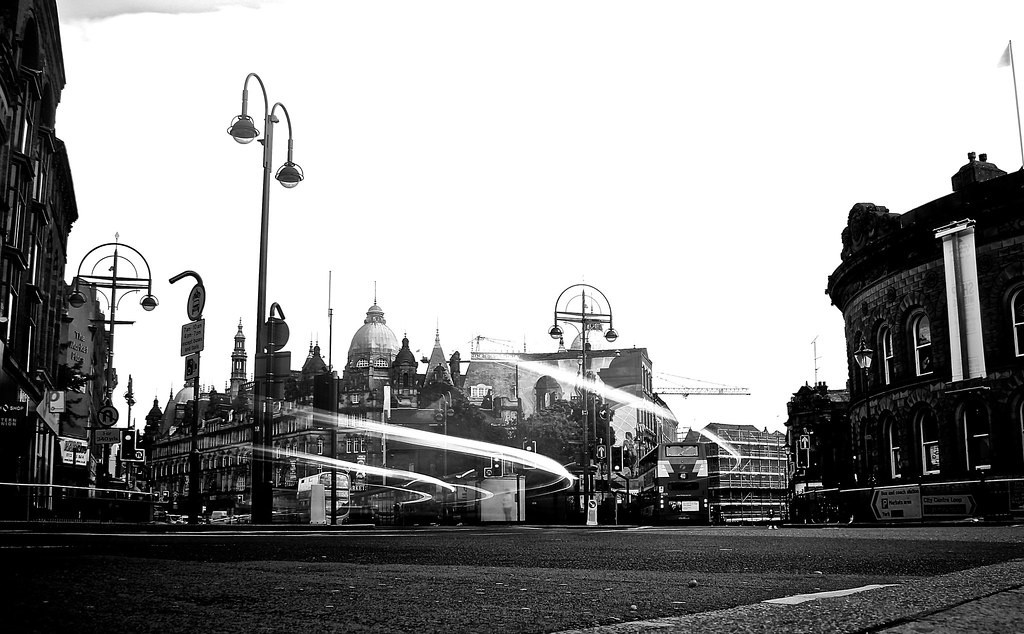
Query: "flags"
998 45 1011 67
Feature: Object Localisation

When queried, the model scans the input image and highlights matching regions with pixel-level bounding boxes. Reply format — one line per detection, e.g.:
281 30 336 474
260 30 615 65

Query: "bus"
181 515 202 525
297 472 351 525
169 515 181 524
211 511 227 525
637 442 709 525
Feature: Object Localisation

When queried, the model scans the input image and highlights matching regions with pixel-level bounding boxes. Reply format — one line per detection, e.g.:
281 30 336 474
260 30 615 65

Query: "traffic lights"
603 481 610 492
120 430 145 462
523 441 537 469
796 440 809 468
484 453 503 478
610 446 623 473
596 480 602 491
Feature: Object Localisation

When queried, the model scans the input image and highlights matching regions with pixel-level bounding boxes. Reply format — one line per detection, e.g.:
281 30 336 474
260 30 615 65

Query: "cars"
224 514 252 524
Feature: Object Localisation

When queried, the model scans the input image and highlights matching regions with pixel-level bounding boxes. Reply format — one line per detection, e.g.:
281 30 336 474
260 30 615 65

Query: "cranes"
420 336 751 397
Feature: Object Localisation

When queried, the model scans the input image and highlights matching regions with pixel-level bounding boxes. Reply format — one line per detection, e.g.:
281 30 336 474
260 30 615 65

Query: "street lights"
434 390 455 526
853 337 875 487
549 284 618 522
67 233 159 498
124 374 136 491
228 73 305 526
781 442 793 524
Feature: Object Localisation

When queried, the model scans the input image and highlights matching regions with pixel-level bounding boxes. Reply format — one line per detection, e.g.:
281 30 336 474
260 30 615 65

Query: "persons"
623 432 637 462
502 488 512 527
